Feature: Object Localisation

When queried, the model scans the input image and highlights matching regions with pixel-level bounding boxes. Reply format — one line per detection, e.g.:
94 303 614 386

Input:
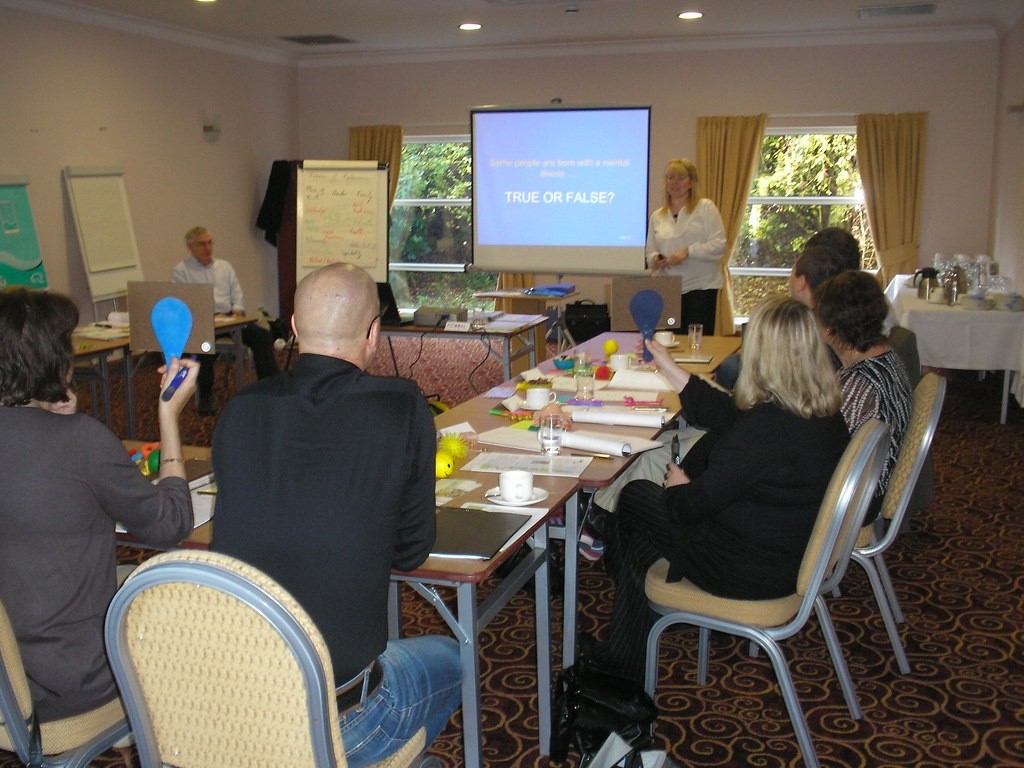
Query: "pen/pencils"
94 324 112 328
633 405 670 411
569 453 616 460
671 435 680 471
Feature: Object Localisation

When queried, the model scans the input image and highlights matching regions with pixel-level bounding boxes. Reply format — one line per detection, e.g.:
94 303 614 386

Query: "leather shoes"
576 491 614 541
576 632 606 668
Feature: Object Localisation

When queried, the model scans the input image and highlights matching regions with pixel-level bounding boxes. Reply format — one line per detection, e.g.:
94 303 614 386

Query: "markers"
161 355 197 402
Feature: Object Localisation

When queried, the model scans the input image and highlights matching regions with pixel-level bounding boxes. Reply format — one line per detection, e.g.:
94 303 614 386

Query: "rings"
663 478 668 486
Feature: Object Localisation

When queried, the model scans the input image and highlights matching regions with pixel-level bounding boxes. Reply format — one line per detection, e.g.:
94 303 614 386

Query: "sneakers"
547 503 606 562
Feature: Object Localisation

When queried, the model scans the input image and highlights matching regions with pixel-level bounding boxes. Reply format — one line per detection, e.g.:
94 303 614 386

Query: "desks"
886 271 1023 424
55 315 259 440
436 331 760 688
474 292 579 347
378 310 544 381
112 440 580 768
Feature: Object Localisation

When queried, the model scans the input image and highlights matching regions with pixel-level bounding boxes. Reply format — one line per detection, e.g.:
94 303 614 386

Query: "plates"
517 400 560 410
486 486 549 506
663 341 680 348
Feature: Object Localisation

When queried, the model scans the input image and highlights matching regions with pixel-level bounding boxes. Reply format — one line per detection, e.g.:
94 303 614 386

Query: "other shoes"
198 396 216 417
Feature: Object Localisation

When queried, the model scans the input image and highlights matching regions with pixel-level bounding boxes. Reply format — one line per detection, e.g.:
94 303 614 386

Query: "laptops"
375 282 414 327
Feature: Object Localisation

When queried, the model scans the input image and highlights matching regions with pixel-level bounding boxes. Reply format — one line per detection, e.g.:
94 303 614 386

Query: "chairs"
103 550 426 768
644 372 947 768
0 603 132 768
215 337 256 394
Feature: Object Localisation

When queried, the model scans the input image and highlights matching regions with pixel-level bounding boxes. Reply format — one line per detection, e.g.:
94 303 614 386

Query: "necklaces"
850 349 859 364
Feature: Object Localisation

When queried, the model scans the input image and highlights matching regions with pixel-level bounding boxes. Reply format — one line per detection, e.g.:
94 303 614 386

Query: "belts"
336 658 383 714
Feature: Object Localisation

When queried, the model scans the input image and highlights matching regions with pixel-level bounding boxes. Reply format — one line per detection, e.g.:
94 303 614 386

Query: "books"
474 353 714 457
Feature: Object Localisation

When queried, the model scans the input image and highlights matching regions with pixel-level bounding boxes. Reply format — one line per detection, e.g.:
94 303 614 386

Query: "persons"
646 158 727 336
577 296 852 692
549 229 914 560
171 227 279 417
206 264 463 768
0 286 200 753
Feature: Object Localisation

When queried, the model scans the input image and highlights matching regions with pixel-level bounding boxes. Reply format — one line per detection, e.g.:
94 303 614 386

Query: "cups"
540 415 562 457
688 324 703 350
526 388 556 408
655 331 675 344
609 354 631 370
576 369 595 402
499 470 534 502
572 348 588 370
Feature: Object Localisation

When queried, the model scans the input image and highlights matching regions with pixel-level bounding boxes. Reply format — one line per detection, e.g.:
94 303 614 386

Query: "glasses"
366 302 389 339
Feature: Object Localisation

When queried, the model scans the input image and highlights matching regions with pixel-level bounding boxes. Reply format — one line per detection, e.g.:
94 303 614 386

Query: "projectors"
414 307 468 327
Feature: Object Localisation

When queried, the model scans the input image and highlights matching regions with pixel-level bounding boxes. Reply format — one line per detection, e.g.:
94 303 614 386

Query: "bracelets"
159 457 185 467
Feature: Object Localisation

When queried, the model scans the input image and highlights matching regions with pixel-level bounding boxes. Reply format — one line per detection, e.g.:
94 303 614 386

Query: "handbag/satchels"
549 658 664 757
565 299 611 339
495 528 565 597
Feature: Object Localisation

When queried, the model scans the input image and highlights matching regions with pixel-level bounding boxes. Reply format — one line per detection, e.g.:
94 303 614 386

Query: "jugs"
913 268 939 300
